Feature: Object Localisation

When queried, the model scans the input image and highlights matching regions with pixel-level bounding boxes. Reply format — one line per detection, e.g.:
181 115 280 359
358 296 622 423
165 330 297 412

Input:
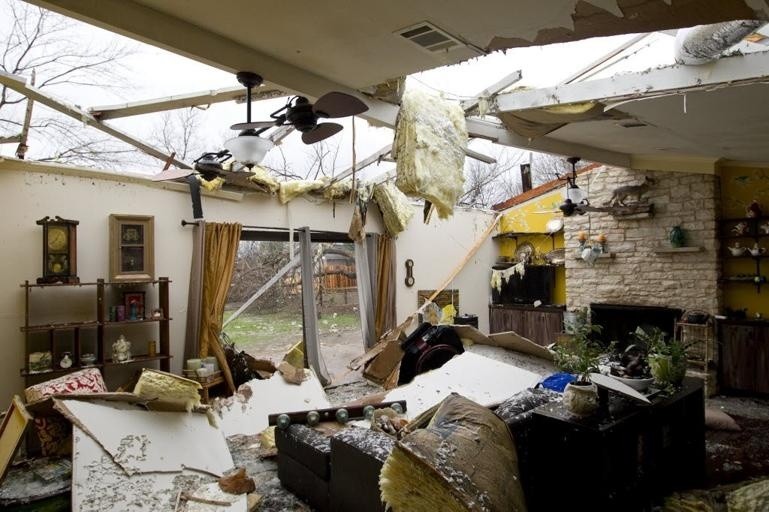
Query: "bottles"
149 340 157 360
669 225 685 248
60 351 73 370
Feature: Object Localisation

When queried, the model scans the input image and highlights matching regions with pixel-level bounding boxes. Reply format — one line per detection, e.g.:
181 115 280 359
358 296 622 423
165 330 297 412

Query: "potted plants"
545 305 709 415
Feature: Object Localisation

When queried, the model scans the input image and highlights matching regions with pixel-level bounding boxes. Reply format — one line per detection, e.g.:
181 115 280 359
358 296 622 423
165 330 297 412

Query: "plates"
515 242 534 261
544 247 565 265
607 371 655 391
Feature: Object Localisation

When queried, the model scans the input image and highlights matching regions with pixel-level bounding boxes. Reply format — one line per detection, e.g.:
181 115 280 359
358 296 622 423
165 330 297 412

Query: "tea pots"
727 221 769 256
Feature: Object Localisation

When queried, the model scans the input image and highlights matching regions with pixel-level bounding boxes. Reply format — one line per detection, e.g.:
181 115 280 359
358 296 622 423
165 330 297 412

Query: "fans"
229 91 370 145
151 149 268 195
530 176 619 216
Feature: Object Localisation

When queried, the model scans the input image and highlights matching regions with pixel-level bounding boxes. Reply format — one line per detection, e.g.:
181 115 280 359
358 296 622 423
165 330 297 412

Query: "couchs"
273 384 562 512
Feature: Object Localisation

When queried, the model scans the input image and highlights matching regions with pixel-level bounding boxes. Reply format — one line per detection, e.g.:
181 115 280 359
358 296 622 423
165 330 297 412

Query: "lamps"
563 157 584 205
223 71 274 174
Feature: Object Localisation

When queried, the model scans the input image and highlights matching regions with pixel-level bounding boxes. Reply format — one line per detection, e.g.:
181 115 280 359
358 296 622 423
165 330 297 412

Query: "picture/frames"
107 211 156 285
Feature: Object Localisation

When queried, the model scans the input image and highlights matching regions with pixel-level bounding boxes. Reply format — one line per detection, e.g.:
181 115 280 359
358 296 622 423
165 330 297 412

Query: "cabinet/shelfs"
533 376 709 511
18 277 174 460
490 303 565 350
719 219 769 400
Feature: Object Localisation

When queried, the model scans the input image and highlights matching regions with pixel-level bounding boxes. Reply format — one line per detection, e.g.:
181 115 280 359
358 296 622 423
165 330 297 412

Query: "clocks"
35 215 80 285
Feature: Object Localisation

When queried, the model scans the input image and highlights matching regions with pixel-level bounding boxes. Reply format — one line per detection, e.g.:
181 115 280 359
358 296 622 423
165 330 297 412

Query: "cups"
151 309 163 320
109 303 137 323
